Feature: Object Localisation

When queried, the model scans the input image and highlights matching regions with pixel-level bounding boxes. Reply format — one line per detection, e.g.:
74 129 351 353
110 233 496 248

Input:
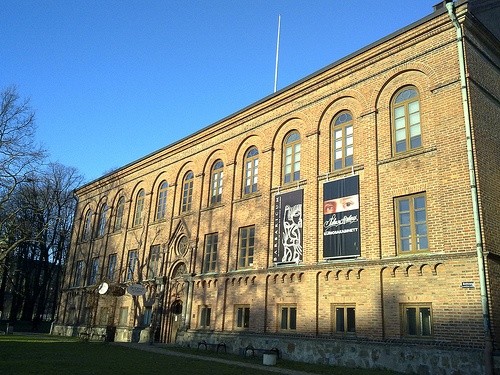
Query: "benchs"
198 340 226 353
80 332 103 342
239 345 280 362
